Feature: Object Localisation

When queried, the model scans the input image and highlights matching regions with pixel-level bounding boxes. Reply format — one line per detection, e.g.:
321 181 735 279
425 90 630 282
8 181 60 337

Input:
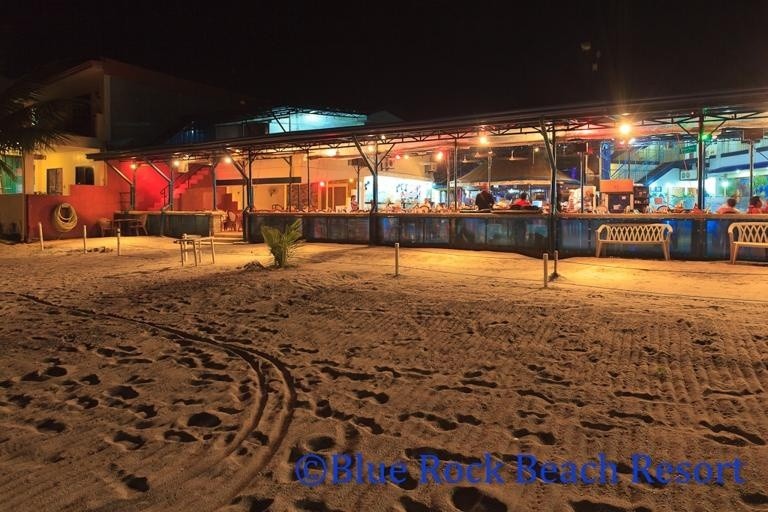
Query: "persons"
747 194 767 214
475 184 496 211
716 197 740 214
350 195 358 211
512 192 532 206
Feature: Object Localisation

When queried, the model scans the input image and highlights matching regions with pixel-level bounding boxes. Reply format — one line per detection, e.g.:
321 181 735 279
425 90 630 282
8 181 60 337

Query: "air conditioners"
381 158 397 171
424 162 438 175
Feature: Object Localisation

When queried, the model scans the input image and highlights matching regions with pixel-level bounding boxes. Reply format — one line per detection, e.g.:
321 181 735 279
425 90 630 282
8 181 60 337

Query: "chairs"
223 209 236 231
175 234 216 267
96 214 149 236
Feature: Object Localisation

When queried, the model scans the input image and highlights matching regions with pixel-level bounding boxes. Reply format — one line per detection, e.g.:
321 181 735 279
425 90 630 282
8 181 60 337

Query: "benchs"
595 221 768 265
